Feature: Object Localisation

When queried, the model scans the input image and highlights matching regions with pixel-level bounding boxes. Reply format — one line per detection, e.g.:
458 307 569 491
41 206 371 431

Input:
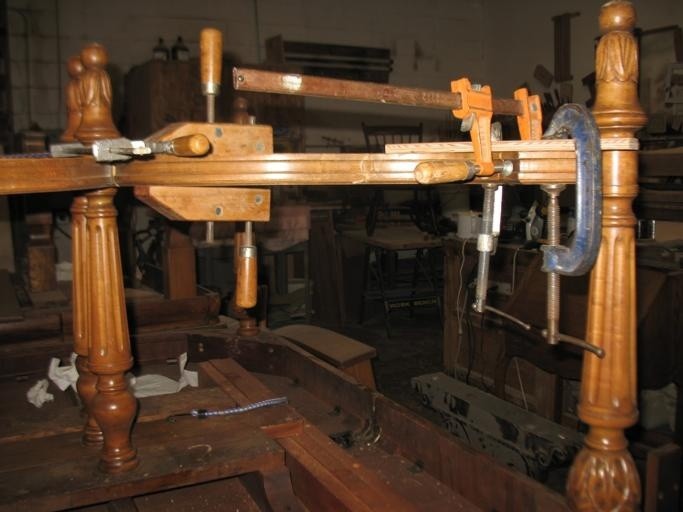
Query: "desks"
340 225 446 341
